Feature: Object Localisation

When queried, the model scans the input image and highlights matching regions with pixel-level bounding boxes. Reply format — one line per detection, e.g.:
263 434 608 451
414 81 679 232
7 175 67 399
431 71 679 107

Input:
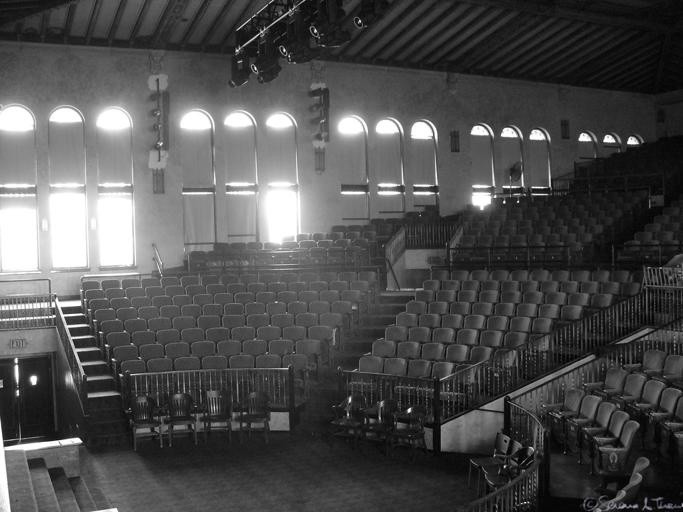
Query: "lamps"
227 0 369 89
149 78 164 164
307 87 330 145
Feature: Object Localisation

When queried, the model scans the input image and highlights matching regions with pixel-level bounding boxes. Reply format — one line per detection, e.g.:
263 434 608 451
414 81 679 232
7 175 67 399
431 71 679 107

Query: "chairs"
83 136 683 512
